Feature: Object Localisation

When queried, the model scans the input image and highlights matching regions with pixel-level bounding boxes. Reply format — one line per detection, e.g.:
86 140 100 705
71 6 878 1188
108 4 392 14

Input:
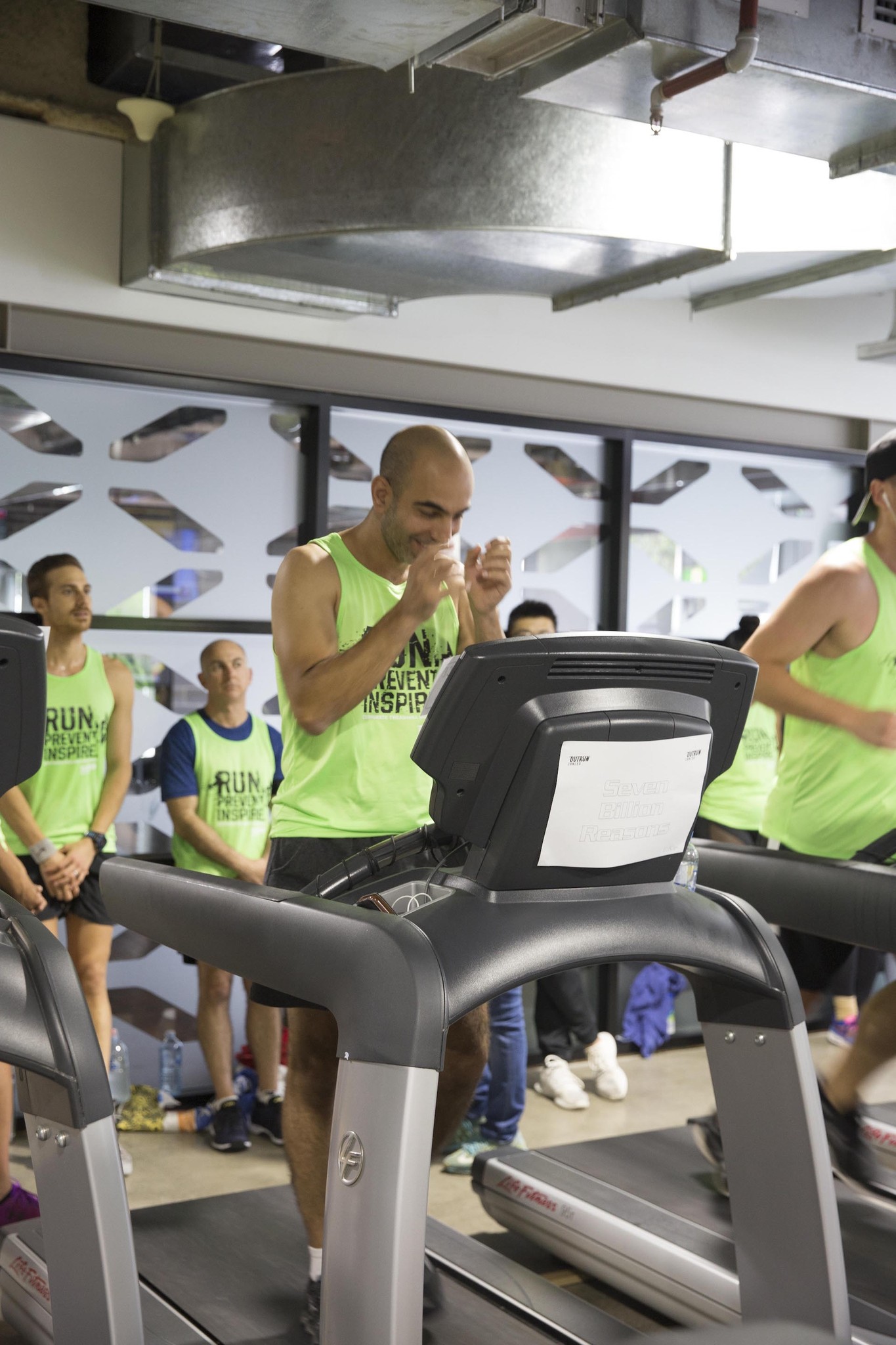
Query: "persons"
787 929 859 1052
689 432 896 1216
246 424 510 1345
695 614 783 846
163 639 282 1150
0 553 132 1237
438 602 629 1175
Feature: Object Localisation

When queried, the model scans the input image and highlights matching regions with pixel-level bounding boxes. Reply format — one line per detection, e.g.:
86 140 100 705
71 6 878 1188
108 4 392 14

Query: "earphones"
882 492 891 507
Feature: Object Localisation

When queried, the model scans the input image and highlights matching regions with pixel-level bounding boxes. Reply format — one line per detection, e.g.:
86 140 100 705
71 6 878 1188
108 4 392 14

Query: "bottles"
673 831 699 892
158 1030 183 1098
109 1027 131 1103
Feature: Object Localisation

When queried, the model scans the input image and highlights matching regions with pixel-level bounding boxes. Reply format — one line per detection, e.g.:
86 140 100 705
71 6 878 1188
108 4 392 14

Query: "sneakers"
687 1107 731 1198
248 1095 287 1144
205 1100 252 1150
0 1177 41 1234
441 1131 481 1153
827 1013 859 1051
816 1076 874 1191
443 1132 529 1175
533 1054 592 1109
585 1030 627 1100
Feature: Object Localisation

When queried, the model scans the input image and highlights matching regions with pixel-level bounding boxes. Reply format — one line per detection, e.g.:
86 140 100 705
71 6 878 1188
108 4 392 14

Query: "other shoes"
305 1275 431 1341
120 1145 132 1174
423 1254 440 1308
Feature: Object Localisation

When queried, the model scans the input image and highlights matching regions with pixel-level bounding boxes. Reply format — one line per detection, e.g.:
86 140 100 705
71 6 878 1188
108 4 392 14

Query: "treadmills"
100 631 860 1344
0 610 147 1345
473 838 896 1344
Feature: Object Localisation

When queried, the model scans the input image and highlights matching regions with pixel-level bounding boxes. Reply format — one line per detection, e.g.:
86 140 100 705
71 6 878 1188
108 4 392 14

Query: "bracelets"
30 839 56 865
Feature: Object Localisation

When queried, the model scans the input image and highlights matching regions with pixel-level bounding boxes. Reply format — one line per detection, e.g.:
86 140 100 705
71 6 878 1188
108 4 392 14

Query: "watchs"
87 832 107 853
